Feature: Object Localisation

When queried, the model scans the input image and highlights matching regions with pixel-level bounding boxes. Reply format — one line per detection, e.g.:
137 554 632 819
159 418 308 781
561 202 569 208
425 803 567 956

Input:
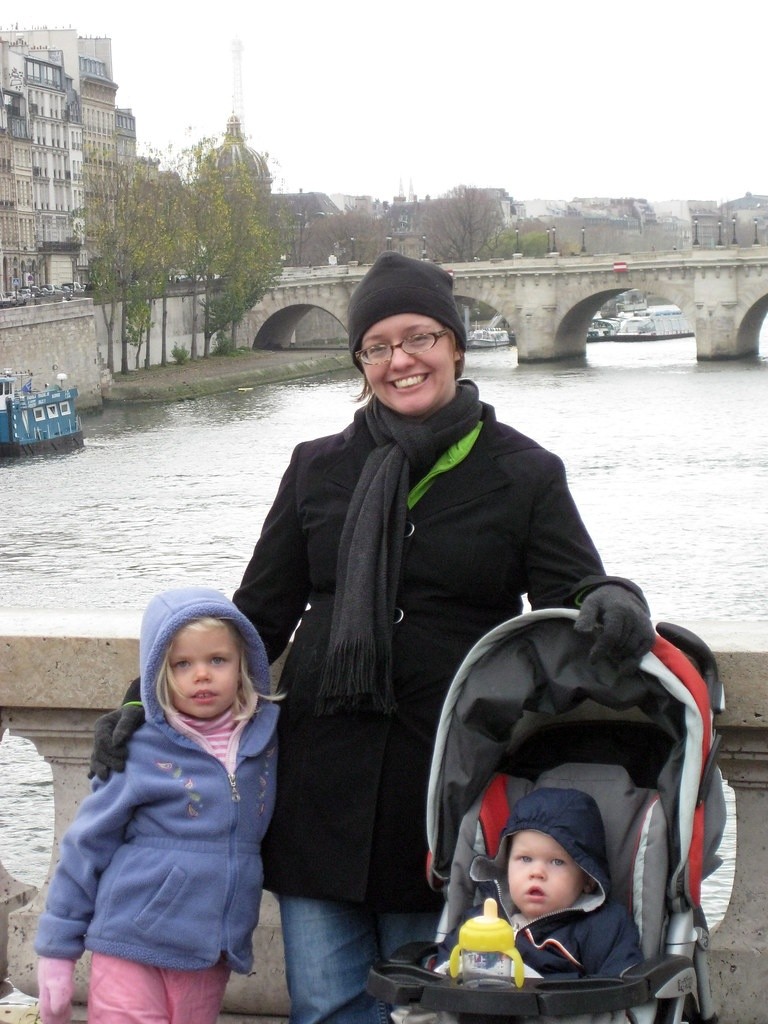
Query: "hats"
346 251 466 374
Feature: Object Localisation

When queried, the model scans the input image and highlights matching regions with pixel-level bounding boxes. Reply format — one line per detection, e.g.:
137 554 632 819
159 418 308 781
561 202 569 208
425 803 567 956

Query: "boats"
586 290 696 340
464 327 510 348
0 366 85 457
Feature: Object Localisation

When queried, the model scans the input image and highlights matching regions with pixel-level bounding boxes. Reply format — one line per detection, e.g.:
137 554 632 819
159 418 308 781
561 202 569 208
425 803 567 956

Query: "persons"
432 786 645 1024
32 584 283 1024
83 252 656 1024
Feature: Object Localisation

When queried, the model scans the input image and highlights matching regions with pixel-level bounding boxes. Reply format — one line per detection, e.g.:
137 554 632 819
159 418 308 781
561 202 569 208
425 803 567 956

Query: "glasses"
355 328 449 365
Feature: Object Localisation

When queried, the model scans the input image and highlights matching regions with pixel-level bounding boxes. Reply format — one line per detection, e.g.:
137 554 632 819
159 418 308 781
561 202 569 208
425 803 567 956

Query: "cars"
0 274 148 305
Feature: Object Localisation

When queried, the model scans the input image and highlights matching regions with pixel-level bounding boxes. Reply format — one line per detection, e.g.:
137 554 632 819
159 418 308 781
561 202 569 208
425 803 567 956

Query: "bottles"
449 898 524 1024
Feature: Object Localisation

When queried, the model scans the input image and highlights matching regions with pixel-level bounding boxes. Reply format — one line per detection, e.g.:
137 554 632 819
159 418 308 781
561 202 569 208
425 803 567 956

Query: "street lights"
693 218 701 245
421 235 427 259
546 229 551 253
731 217 738 244
351 237 355 261
717 220 723 245
581 225 587 252
515 228 519 253
552 226 558 252
754 218 760 244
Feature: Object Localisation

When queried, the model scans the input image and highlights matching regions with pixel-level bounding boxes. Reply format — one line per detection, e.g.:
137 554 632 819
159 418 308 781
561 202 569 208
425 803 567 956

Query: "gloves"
87 704 145 781
38 957 74 1024
573 584 656 670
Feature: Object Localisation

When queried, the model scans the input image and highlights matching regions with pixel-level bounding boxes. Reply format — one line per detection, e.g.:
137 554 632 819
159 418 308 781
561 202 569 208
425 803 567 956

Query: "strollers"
368 608 743 1024
